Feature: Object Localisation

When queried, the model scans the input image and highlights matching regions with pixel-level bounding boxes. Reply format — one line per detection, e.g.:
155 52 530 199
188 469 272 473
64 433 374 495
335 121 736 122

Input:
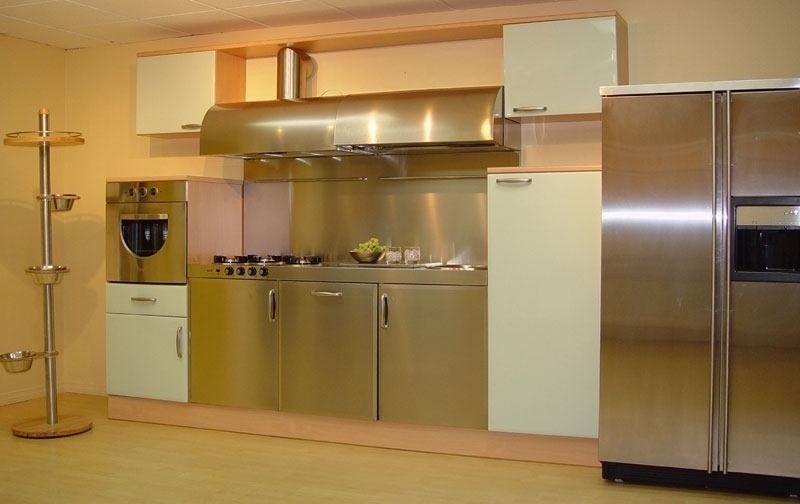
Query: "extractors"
198 46 521 164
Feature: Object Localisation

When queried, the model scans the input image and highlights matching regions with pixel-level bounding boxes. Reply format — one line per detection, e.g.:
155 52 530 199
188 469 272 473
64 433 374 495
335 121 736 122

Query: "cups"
404 247 420 264
387 247 402 264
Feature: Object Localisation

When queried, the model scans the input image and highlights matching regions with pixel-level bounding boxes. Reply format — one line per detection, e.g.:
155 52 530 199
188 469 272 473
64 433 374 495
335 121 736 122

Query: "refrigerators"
600 75 799 501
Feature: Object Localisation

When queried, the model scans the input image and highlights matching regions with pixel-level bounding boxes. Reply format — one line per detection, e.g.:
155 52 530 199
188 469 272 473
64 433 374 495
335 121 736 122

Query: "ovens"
105 175 245 286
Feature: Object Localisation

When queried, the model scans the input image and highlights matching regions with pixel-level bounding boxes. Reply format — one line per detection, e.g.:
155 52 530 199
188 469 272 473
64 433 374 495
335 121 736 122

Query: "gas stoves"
188 253 321 281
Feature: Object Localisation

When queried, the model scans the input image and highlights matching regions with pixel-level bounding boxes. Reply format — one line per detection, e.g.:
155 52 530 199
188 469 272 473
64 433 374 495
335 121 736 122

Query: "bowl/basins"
51 193 77 212
349 250 386 263
0 350 36 374
27 264 67 285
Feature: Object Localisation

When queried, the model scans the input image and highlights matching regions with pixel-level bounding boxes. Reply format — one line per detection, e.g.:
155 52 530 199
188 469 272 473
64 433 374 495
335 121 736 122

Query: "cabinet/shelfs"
134 49 247 135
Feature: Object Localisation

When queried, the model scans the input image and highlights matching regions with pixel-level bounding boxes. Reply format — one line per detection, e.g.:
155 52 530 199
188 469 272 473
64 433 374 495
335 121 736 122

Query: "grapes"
353 238 386 254
